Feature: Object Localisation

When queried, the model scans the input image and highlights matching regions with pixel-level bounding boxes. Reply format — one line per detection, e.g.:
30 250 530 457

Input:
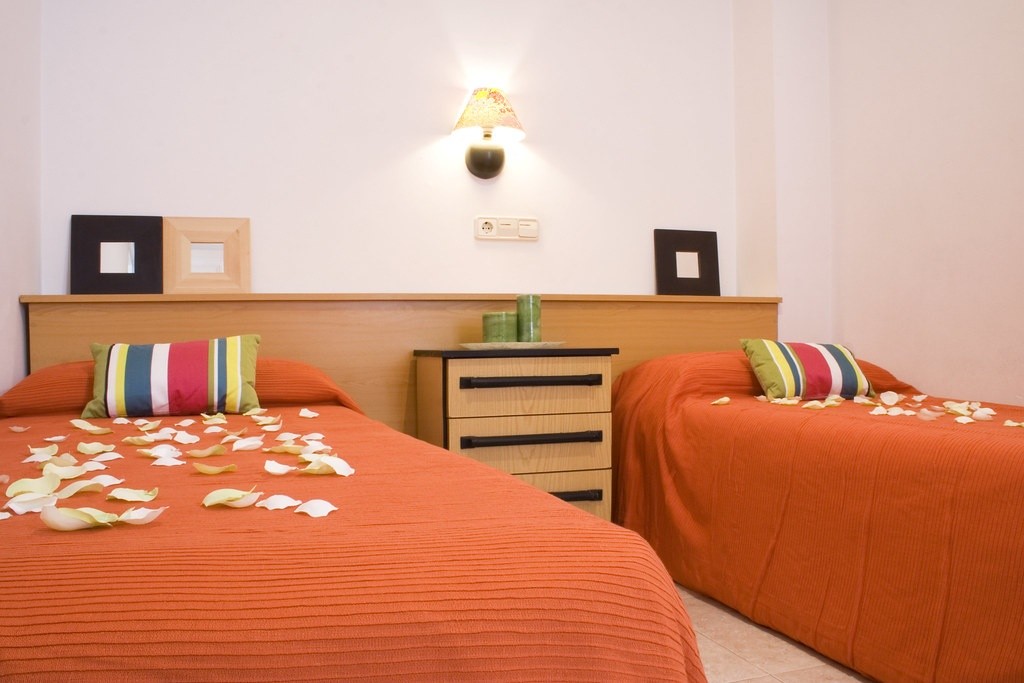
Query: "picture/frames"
654 229 721 296
163 215 252 294
69 215 163 294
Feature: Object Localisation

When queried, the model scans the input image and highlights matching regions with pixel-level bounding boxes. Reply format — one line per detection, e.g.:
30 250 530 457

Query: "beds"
612 349 1024 683
0 358 706 682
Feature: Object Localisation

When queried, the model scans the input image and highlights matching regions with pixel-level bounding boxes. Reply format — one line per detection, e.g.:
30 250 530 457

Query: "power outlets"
473 216 541 242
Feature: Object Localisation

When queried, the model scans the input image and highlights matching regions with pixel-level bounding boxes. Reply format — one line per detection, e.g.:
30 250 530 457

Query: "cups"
482 310 518 342
517 294 541 342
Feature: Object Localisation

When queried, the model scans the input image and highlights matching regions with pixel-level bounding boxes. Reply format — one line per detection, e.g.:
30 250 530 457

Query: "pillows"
739 338 876 401
80 333 262 418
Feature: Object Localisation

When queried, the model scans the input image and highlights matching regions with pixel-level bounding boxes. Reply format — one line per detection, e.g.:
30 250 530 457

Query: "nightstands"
414 346 620 521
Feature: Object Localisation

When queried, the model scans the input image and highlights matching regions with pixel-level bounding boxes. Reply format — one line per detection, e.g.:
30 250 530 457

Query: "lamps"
450 89 525 179
